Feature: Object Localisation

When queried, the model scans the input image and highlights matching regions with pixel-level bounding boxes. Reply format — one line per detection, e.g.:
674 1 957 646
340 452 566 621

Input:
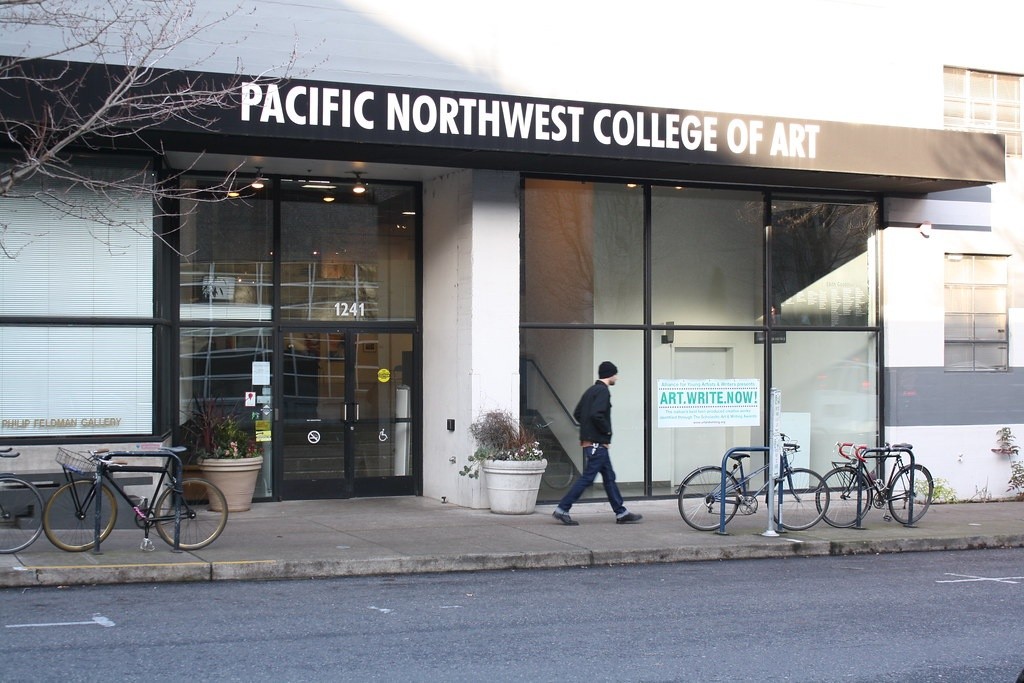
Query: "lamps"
352 172 365 193
252 166 265 188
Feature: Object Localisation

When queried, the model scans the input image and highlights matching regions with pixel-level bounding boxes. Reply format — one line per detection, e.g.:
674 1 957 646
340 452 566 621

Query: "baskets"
784 439 798 455
58 448 95 473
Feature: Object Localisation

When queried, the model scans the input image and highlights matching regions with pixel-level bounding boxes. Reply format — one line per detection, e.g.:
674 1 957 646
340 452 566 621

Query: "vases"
481 459 547 513
197 455 263 512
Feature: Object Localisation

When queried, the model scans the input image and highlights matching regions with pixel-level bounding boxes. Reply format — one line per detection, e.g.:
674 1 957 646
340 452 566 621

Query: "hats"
598 361 617 379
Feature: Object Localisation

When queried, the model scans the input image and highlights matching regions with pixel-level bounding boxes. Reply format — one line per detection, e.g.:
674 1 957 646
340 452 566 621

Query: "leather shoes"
616 512 643 524
553 510 577 524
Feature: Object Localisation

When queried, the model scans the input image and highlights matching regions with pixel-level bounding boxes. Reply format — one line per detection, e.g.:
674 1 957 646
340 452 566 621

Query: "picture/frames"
364 343 377 352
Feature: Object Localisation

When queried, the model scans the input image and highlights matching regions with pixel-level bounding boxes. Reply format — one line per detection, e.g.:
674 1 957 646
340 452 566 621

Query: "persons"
553 362 643 525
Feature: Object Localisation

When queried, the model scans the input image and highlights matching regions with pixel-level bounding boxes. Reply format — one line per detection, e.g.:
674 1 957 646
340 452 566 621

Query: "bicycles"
0 447 48 556
675 432 831 532
815 442 935 529
41 447 229 553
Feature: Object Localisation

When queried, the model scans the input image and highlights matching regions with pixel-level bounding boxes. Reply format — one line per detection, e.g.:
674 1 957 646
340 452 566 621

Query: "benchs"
0 471 153 530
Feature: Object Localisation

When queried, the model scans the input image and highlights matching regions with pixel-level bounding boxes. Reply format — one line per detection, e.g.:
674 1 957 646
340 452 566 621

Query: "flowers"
459 410 544 478
180 389 265 460
